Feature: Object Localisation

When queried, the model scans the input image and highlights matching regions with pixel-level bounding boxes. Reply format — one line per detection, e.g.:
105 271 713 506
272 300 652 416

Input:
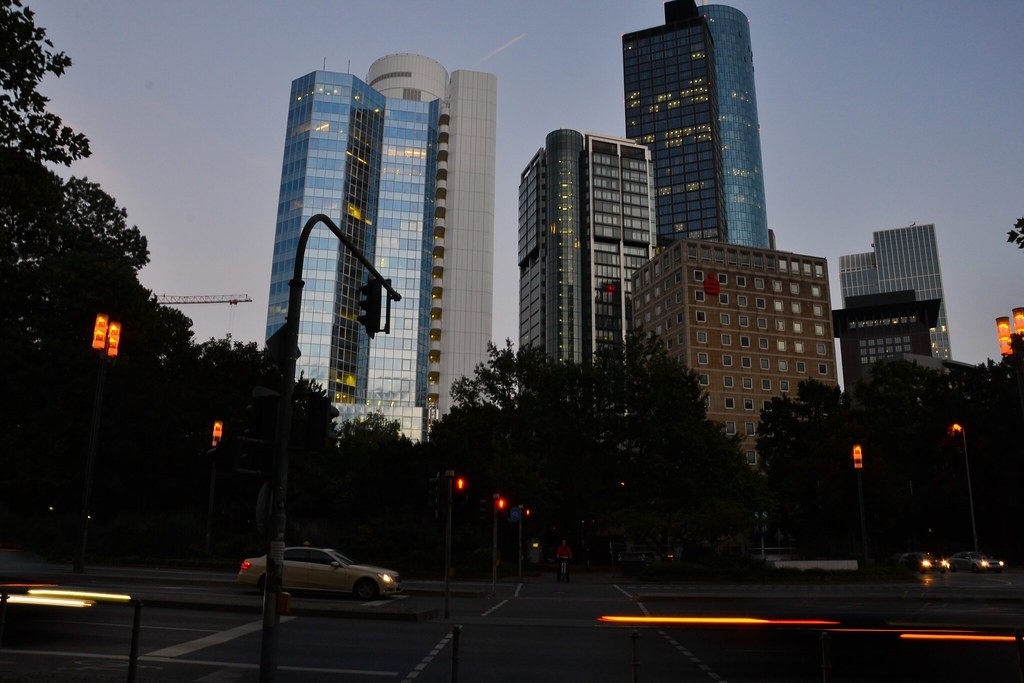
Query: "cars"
899 551 949 574
946 552 1006 574
237 547 405 602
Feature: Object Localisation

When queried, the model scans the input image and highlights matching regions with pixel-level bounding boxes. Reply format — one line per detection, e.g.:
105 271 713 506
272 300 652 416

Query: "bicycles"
558 556 570 584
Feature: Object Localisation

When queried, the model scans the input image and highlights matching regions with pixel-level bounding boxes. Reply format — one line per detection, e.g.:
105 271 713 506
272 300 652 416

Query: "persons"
556 540 572 583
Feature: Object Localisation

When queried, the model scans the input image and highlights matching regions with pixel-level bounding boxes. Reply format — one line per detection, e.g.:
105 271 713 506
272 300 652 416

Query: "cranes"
148 292 253 304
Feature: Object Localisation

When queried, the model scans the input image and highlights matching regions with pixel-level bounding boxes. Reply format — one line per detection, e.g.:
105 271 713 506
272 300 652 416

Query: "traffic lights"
107 322 120 356
93 315 108 350
213 422 222 446
854 446 863 468
358 279 383 337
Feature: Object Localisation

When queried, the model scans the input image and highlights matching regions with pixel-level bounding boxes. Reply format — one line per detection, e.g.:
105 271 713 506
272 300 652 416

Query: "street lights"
953 423 978 551
